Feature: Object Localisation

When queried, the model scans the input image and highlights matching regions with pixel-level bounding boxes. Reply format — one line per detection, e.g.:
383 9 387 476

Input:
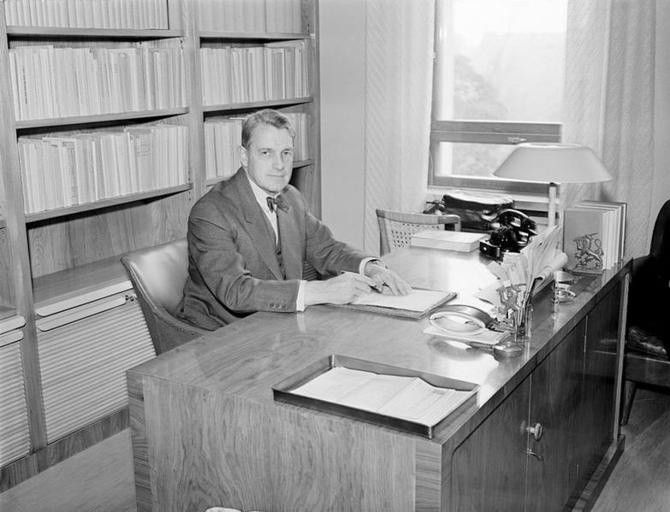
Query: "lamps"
490 140 614 229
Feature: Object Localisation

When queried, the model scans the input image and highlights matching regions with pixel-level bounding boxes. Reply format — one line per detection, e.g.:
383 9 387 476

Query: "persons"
173 108 414 330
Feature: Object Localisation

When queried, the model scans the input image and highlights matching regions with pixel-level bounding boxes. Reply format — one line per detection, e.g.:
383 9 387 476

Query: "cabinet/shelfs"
1 0 323 505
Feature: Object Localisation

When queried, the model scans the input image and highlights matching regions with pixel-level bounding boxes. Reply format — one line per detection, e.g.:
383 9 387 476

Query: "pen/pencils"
519 278 537 337
341 270 382 293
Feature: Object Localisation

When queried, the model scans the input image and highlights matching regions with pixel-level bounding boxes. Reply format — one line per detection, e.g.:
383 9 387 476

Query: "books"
563 201 627 275
411 229 488 253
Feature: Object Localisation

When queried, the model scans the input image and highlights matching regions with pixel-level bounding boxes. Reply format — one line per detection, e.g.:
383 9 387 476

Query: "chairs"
376 207 462 250
116 235 220 354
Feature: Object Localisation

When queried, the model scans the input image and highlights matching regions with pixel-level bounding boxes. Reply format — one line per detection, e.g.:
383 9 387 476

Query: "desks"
125 246 641 512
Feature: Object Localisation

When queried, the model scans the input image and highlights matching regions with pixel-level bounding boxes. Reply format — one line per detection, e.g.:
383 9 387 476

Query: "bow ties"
267 195 289 212
